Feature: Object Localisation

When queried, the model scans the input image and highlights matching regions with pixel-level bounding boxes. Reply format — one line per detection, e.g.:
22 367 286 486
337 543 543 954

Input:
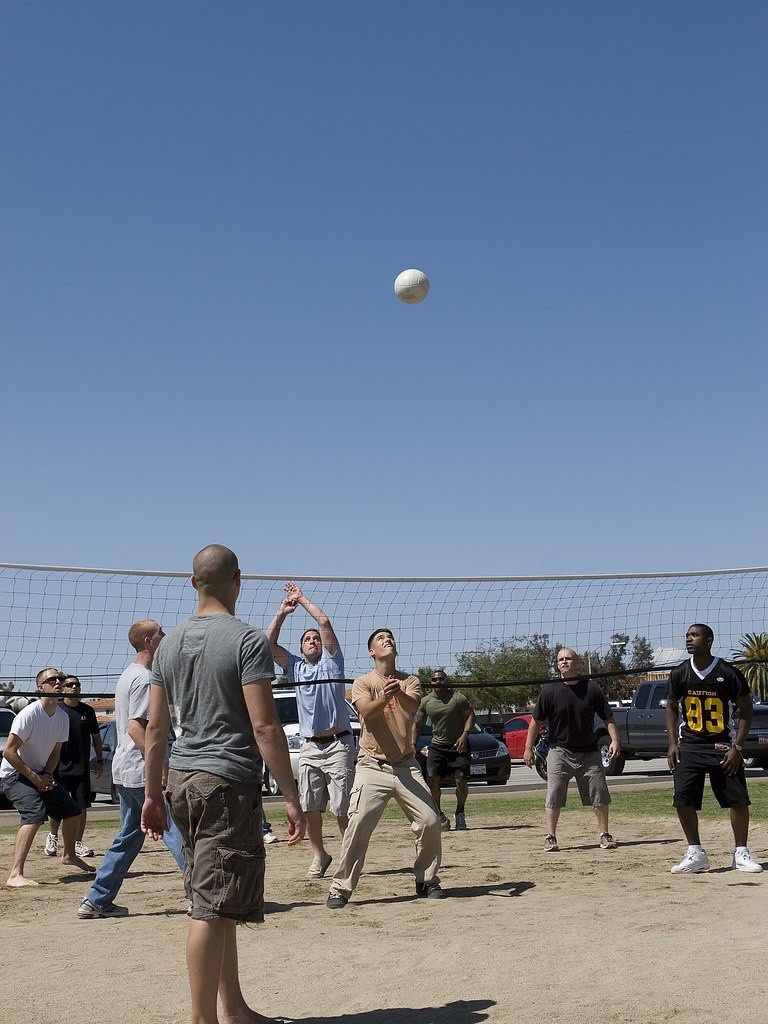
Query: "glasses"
62 682 81 687
41 677 64 684
431 677 446 682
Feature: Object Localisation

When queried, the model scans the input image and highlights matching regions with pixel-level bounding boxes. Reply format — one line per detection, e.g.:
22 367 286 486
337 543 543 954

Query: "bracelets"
44 772 51 776
733 744 743 751
97 760 104 764
411 743 416 745
463 731 470 734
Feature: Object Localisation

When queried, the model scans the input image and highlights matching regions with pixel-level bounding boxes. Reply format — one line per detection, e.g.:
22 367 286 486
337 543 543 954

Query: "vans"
260 689 362 797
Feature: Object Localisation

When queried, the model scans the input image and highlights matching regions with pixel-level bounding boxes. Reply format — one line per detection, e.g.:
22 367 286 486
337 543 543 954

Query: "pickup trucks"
593 680 768 776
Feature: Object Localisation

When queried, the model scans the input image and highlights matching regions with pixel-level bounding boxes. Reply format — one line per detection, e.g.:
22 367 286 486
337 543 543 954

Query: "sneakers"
78 896 129 917
327 891 348 909
416 881 444 899
544 835 558 852
442 817 451 830
307 851 332 878
262 831 277 843
732 849 764 872
671 848 710 872
76 840 94 856
454 812 466 830
45 831 58 856
599 831 616 849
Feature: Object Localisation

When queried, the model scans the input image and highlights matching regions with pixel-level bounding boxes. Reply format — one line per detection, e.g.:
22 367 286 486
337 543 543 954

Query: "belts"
306 730 351 743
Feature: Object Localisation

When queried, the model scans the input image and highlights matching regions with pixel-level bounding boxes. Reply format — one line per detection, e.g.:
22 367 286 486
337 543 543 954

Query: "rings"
46 786 48 788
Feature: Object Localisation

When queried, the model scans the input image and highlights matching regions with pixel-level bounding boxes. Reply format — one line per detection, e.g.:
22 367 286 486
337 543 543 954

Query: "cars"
395 713 512 785
0 707 17 808
89 720 121 804
500 714 549 760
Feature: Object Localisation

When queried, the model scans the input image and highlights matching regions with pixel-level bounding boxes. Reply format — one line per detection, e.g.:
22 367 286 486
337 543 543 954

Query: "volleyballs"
394 268 430 304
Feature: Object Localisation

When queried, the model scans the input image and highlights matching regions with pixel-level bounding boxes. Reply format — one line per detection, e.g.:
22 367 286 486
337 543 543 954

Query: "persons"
524 648 620 851
410 670 476 831
0 668 97 886
140 544 306 1024
266 581 356 878
665 624 763 872
77 619 184 914
44 675 103 857
326 629 445 909
